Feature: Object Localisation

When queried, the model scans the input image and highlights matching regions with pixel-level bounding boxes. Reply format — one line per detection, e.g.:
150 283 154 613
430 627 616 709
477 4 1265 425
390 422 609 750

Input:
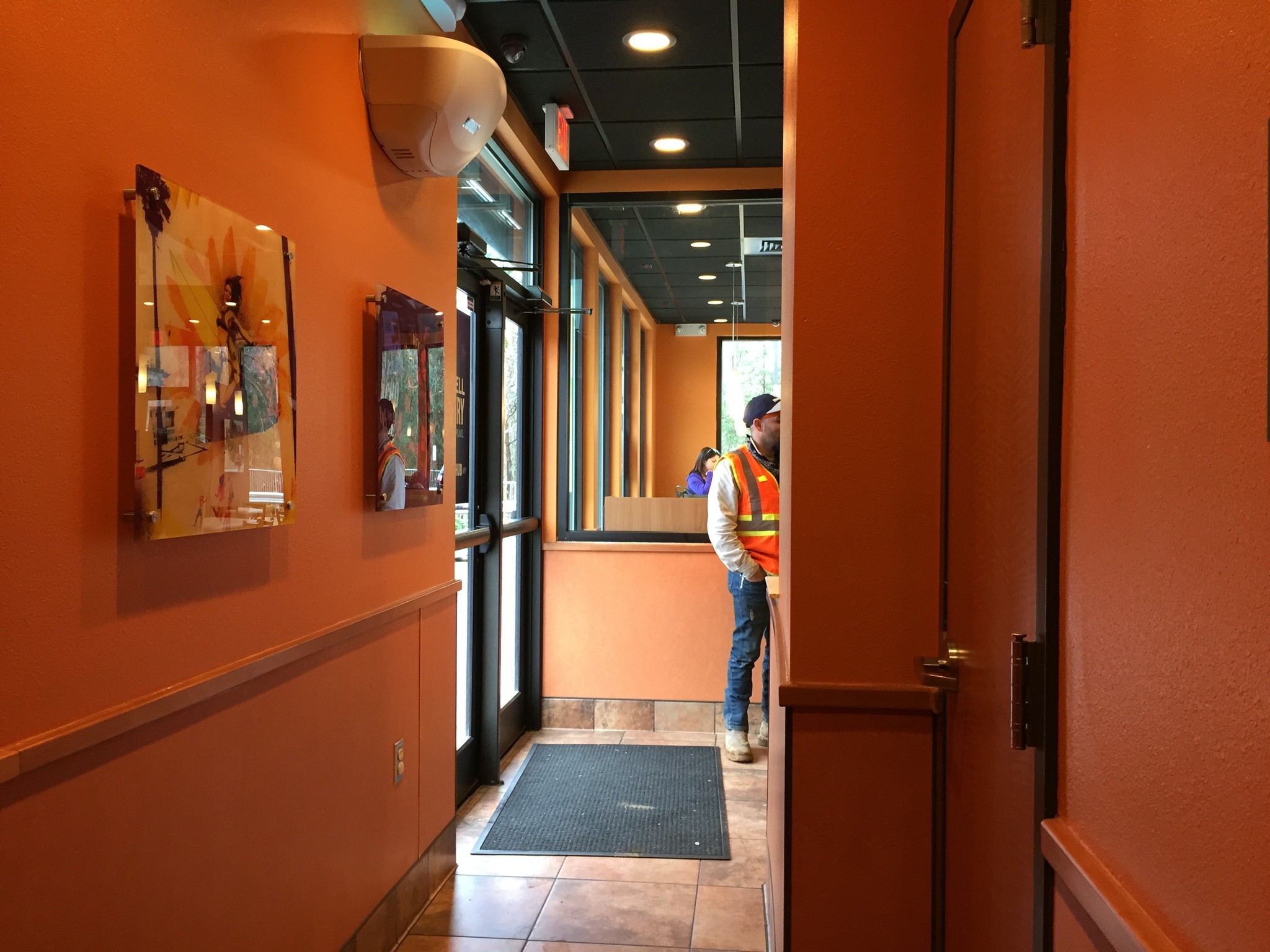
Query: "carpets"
469 744 731 860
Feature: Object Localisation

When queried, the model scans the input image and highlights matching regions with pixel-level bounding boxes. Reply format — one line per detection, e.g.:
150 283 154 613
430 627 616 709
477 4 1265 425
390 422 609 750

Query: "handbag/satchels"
675 485 696 498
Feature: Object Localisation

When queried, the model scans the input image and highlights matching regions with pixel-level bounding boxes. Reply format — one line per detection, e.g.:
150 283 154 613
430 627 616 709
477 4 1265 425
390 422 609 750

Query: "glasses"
706 448 720 455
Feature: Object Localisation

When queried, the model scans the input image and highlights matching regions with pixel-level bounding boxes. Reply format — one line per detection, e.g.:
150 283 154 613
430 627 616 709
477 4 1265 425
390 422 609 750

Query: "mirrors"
556 186 782 551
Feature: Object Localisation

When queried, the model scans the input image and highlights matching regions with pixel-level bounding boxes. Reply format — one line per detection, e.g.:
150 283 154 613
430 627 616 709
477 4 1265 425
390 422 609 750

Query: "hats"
742 393 780 426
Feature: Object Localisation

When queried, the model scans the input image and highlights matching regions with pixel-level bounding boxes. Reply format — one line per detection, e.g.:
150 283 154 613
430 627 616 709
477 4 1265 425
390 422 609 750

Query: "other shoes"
725 729 753 762
757 722 769 747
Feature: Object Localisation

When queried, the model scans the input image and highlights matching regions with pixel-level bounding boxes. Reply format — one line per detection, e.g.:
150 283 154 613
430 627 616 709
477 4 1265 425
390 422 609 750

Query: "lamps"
725 260 744 373
675 323 707 337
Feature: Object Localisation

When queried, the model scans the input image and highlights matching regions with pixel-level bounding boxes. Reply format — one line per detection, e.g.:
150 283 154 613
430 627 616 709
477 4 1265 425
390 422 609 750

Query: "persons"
686 447 720 495
706 393 782 761
140 391 223 535
376 398 406 511
215 275 258 408
